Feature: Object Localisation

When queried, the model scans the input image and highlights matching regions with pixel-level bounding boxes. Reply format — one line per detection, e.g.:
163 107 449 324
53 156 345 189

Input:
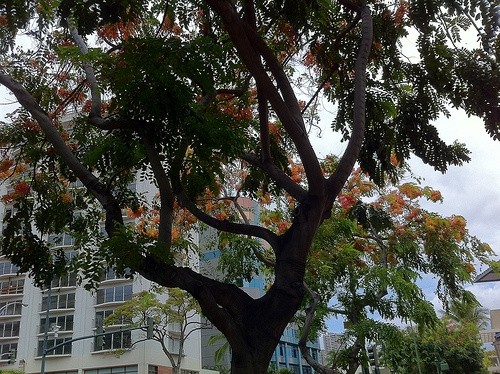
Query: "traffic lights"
146 317 155 339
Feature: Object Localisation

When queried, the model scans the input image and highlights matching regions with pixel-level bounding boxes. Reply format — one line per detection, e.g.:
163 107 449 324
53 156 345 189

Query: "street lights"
40 245 75 374
